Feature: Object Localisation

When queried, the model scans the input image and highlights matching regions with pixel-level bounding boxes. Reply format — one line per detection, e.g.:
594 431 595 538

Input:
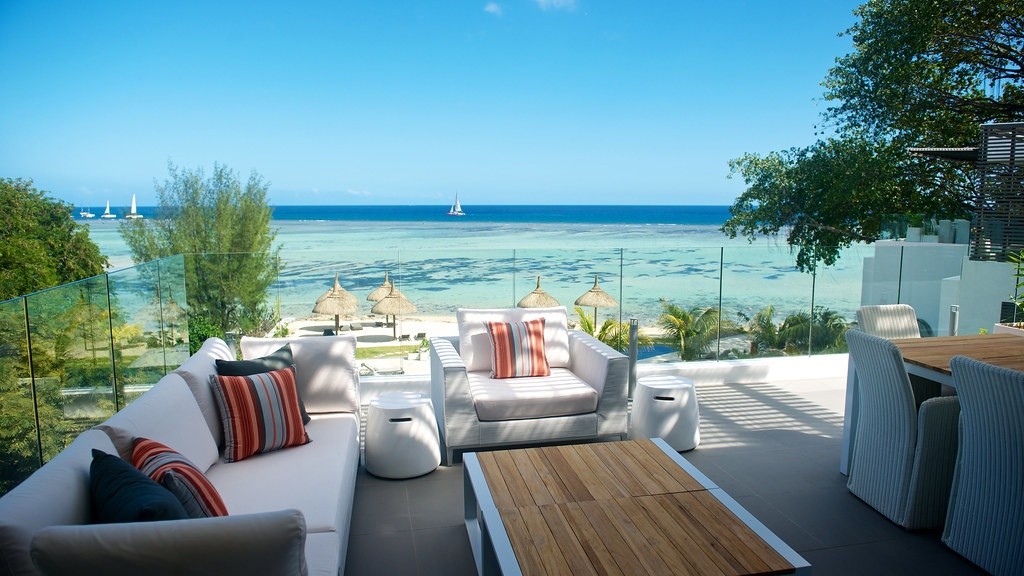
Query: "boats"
80 212 95 219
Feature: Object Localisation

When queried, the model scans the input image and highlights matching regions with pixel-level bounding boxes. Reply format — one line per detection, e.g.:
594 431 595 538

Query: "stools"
630 375 700 452
364 391 442 480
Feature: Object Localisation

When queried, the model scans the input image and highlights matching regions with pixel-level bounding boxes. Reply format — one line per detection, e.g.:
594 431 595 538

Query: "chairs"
856 304 921 340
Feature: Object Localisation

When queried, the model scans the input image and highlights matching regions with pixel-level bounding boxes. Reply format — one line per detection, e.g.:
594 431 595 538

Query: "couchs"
941 356 1024 576
845 328 961 529
0 335 364 576
429 305 630 467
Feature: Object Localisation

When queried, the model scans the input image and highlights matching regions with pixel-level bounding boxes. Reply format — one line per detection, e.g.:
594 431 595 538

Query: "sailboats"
448 192 466 215
101 201 116 218
126 192 144 218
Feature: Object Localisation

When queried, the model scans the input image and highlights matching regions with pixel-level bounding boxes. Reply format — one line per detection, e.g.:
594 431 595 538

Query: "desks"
882 333 1024 398
462 437 813 576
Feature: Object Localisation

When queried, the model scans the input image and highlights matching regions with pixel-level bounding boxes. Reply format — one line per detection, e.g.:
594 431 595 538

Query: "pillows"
482 316 551 378
88 424 230 525
209 343 313 463
